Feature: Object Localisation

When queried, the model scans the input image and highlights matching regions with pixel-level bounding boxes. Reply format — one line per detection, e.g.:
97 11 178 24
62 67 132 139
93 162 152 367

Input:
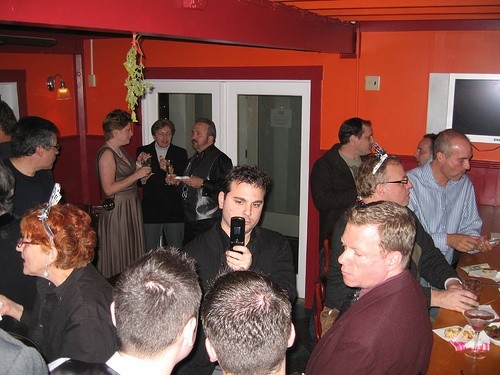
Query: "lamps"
47 73 69 93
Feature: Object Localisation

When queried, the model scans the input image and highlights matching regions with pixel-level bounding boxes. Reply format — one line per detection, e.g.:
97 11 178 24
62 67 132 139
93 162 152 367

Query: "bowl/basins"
485 321 500 346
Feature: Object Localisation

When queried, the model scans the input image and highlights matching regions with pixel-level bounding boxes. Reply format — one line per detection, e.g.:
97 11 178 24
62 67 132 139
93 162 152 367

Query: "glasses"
383 177 409 184
45 144 61 151
16 237 41 248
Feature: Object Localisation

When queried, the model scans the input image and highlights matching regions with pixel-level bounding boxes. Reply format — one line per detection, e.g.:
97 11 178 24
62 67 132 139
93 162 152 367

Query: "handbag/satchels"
102 198 115 211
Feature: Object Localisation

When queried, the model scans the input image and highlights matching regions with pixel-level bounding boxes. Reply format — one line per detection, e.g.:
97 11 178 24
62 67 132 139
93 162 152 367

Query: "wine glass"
141 158 151 180
167 159 176 186
462 309 496 361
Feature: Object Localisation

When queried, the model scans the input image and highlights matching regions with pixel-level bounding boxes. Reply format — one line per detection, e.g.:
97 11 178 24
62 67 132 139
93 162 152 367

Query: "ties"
333 289 360 327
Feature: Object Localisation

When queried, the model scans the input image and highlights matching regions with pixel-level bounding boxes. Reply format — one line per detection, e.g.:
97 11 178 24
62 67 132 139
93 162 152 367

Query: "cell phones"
227 215 248 254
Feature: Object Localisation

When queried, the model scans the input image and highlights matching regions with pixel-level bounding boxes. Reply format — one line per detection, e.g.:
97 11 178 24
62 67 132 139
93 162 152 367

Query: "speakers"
449 73 500 145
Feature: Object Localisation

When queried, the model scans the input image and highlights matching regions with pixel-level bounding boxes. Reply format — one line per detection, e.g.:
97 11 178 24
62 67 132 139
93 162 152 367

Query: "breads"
444 327 473 340
482 268 497 273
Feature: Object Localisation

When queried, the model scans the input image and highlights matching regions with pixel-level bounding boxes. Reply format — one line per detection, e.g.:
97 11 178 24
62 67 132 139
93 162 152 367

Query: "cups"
463 278 481 303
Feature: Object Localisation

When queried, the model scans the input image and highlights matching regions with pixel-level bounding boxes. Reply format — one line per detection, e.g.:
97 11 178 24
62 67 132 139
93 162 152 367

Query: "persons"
306 116 374 354
0 183 120 365
199 269 296 374
0 101 18 167
47 245 203 375
414 132 438 167
95 109 155 279
3 115 66 218
320 152 480 340
305 199 435 375
172 164 298 374
404 128 499 320
165 117 234 247
136 118 189 253
0 158 39 334
